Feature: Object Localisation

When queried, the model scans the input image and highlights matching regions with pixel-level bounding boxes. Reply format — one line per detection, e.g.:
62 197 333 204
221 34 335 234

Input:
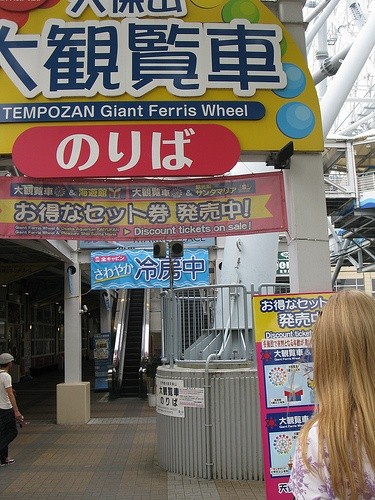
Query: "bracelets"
14 409 19 412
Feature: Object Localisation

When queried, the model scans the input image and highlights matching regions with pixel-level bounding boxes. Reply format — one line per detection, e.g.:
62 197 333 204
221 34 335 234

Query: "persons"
0 353 23 466
287 289 375 500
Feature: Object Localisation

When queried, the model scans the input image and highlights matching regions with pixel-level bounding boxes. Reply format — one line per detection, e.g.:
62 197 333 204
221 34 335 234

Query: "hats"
0 353 14 364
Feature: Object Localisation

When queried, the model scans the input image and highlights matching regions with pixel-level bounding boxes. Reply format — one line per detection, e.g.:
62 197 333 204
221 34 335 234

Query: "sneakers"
1 459 15 467
